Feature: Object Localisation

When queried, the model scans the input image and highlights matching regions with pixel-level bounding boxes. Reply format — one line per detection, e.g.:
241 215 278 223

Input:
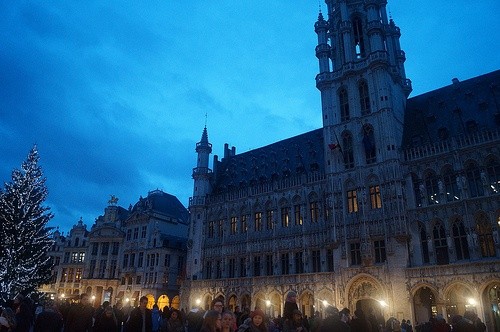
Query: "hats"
251 310 265 319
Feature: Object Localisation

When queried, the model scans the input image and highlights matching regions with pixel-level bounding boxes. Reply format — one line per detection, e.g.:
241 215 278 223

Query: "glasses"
213 305 224 308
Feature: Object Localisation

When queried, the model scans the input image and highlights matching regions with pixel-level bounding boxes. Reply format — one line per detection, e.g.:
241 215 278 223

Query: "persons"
0 292 489 332
